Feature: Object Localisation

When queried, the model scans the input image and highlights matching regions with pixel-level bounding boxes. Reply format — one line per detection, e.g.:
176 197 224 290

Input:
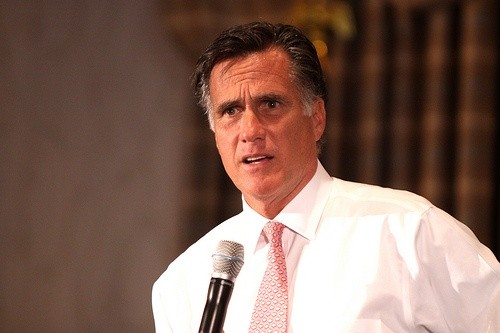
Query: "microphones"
198 240 245 333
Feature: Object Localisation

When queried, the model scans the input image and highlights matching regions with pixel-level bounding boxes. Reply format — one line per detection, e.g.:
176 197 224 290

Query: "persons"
152 22 500 333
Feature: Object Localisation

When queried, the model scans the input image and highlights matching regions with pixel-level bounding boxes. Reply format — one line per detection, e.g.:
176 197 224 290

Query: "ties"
248 221 288 333
